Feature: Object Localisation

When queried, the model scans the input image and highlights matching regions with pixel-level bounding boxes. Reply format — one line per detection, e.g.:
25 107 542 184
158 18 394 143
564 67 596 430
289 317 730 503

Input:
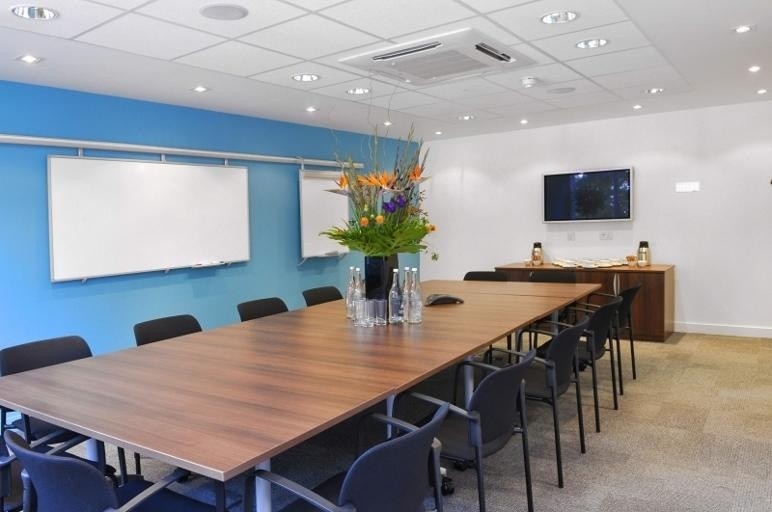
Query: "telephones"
424 293 464 306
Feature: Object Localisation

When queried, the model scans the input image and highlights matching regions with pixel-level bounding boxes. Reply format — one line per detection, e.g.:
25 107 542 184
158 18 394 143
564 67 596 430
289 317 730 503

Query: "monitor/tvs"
542 167 633 224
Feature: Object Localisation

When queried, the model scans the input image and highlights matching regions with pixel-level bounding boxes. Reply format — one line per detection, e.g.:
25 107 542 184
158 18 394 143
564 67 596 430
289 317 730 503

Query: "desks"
2 280 601 510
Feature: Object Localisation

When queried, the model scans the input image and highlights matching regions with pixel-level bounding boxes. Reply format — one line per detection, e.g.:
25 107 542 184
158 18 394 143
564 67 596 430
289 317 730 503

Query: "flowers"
315 121 442 262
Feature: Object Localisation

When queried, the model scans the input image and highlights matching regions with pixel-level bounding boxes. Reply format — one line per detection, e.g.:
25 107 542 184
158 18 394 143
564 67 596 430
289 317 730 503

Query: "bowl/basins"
625 255 648 268
523 258 541 267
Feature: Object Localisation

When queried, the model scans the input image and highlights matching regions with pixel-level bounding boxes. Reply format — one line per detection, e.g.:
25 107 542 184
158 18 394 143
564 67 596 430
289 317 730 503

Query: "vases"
363 252 401 323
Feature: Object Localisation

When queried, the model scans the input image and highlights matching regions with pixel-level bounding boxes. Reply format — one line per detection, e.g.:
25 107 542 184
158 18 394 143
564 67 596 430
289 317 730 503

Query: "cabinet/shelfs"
494 259 580 336
574 262 678 343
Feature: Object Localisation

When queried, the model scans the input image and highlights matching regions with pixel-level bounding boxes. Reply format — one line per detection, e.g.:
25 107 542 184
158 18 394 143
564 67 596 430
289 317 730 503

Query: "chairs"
1 336 125 508
133 314 204 484
237 297 290 324
3 431 216 511
484 315 586 489
387 347 536 508
490 270 642 433
246 402 449 510
303 285 345 307
1 269 243 512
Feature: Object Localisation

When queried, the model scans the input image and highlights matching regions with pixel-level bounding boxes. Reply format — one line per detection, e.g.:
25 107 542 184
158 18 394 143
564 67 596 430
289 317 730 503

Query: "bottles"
389 268 423 326
532 242 543 267
345 267 364 318
637 241 651 267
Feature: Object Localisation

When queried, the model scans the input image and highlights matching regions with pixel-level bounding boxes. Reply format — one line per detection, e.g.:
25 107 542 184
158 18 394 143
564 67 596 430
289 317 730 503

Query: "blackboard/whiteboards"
46 154 250 284
299 170 350 258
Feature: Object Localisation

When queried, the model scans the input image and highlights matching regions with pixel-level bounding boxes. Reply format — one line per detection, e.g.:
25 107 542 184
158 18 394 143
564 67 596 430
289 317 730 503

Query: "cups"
350 298 387 329
550 255 622 268
